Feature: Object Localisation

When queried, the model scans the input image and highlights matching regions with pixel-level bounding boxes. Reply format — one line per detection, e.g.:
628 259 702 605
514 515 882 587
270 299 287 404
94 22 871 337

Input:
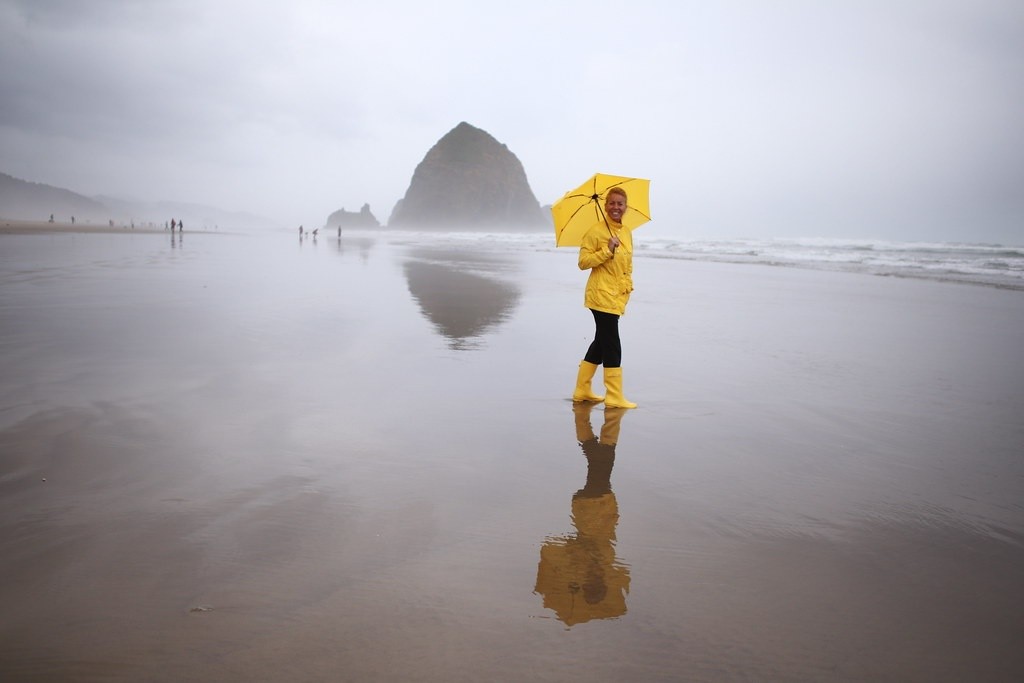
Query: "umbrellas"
550 172 651 248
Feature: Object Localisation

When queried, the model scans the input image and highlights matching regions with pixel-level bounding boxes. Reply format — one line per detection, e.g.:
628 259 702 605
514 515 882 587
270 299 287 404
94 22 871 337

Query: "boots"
573 359 605 403
572 400 596 441
602 367 637 409
600 406 628 447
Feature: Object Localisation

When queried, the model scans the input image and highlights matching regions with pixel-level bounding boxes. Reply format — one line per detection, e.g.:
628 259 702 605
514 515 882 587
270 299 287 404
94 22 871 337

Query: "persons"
299 225 302 234
171 218 175 230
72 216 74 223
573 187 638 408
338 225 341 236
50 214 53 220
165 220 168 231
313 228 318 233
179 220 183 230
